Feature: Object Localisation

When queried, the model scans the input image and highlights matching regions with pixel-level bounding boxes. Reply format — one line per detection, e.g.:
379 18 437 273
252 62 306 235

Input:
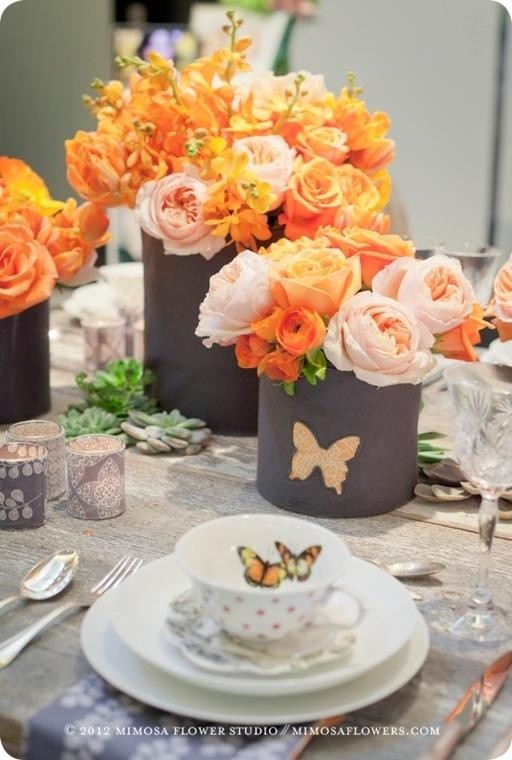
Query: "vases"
0 298 50 423
256 366 421 519
140 226 291 436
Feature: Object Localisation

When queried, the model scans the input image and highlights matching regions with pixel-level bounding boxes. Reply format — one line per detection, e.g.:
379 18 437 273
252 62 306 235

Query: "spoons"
350 554 445 579
0 549 82 608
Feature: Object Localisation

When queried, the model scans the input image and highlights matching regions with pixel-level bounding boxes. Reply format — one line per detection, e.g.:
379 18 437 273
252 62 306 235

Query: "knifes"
415 647 511 760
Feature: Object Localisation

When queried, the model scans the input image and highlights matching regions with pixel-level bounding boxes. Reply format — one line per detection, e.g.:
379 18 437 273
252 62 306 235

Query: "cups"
49 257 145 377
407 234 501 309
64 434 128 521
0 442 50 530
173 514 368 645
6 419 67 504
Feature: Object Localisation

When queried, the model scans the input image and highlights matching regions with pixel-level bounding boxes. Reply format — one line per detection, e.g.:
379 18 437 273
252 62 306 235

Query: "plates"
81 552 432 727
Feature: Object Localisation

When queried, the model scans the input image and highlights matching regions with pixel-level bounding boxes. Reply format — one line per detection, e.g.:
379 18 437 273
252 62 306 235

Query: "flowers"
63 11 396 260
0 155 113 320
193 225 512 395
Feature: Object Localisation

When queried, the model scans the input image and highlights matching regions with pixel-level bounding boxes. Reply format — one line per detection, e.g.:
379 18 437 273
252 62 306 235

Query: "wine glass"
434 361 511 647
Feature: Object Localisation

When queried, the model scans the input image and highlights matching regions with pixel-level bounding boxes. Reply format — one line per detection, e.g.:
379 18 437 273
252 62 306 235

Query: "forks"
0 554 145 670
